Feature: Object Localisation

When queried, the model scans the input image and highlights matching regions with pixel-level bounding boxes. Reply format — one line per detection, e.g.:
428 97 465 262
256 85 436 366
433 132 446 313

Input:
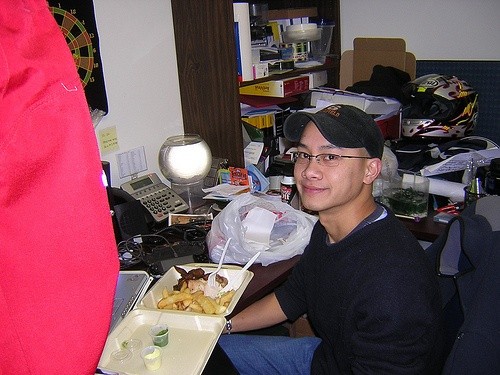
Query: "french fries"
156 282 237 315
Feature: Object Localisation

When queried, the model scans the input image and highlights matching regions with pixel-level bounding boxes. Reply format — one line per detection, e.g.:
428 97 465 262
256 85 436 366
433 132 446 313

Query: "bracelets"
227 319 232 334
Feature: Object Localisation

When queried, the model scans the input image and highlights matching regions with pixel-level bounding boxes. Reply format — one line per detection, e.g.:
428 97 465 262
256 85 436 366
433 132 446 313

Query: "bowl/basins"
97 263 254 375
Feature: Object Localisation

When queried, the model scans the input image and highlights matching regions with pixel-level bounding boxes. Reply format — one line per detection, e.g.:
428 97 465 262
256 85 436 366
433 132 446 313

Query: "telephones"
106 173 189 234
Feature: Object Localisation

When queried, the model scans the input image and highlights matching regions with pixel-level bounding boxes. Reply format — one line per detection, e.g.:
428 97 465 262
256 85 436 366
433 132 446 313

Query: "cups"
140 346 162 370
281 24 335 68
148 324 169 346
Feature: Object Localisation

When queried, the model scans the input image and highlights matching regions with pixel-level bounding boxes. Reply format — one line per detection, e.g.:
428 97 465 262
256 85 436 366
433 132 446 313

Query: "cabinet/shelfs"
171 0 342 170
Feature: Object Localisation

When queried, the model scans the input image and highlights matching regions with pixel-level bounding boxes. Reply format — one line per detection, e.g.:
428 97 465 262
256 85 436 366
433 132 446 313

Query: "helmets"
401 74 479 138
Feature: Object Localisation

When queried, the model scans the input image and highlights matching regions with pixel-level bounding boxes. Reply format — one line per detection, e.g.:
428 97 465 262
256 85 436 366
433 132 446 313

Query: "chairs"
426 195 500 375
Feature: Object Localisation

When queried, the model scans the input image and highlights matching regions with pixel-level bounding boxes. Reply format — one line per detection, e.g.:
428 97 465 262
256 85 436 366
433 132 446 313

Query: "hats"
283 104 389 160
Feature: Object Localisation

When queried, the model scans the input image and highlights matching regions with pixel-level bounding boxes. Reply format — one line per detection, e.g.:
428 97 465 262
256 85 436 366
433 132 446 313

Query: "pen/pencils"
469 176 482 198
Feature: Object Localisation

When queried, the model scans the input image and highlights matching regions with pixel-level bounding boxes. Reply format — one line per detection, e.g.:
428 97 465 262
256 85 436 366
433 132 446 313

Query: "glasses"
290 151 373 167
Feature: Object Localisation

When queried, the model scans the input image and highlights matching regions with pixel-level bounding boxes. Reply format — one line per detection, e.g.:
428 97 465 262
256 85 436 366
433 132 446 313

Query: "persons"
217 104 442 375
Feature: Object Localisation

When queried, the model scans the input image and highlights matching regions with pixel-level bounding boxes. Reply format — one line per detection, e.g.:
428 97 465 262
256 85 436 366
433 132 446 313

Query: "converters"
155 254 195 275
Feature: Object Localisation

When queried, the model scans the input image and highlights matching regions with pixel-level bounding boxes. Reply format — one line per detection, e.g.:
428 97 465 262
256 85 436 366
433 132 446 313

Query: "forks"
221 251 260 291
208 238 232 286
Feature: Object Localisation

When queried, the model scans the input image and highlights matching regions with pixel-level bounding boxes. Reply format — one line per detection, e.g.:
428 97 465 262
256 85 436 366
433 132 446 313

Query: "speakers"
113 200 150 245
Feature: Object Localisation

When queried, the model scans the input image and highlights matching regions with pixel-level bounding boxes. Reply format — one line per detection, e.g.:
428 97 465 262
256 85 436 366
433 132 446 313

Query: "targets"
47 0 110 116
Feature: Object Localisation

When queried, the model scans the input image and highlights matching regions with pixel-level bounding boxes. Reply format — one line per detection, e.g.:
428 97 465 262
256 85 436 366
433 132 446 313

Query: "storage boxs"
239 6 402 139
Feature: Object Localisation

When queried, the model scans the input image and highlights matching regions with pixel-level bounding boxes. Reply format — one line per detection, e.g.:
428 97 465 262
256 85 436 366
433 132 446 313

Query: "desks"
398 195 447 242
122 192 303 320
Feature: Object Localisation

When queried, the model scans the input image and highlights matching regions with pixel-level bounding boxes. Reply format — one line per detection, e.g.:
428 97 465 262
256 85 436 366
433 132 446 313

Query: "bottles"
280 176 296 206
